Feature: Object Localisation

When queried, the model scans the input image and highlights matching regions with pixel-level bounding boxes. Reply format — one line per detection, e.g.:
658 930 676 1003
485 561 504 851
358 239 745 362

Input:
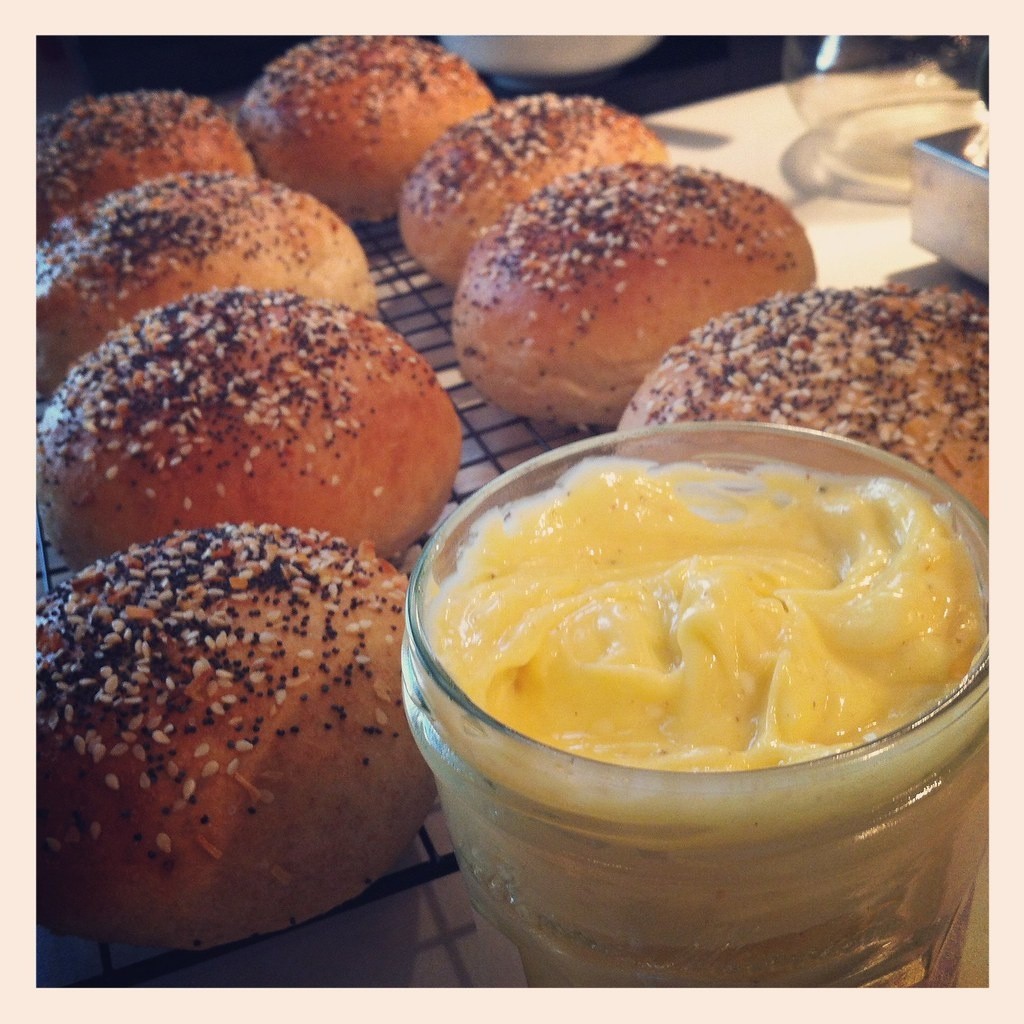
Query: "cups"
780 35 986 195
401 420 989 989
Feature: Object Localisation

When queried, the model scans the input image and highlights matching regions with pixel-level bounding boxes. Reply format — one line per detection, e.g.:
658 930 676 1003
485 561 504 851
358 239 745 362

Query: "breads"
36 35 989 987
